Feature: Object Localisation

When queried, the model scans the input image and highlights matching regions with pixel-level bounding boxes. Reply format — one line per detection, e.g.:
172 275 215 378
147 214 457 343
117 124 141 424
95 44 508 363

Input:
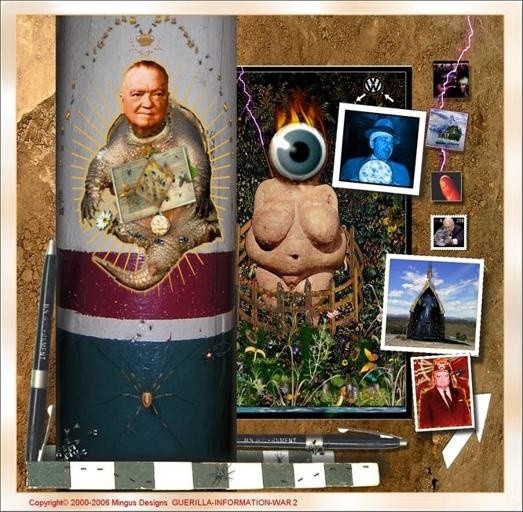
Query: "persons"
118 60 171 138
341 119 412 187
247 177 347 308
420 362 471 428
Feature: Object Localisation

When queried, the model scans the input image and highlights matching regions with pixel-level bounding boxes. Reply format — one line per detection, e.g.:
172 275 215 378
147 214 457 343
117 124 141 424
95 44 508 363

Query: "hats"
434 362 451 373
365 119 401 145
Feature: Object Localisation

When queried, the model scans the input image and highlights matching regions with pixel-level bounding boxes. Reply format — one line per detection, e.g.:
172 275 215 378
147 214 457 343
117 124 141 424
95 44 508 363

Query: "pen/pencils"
21 237 57 462
234 426 409 450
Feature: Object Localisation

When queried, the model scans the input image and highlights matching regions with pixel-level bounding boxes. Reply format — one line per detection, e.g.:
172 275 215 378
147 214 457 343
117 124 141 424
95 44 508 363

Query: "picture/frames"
236 64 413 420
